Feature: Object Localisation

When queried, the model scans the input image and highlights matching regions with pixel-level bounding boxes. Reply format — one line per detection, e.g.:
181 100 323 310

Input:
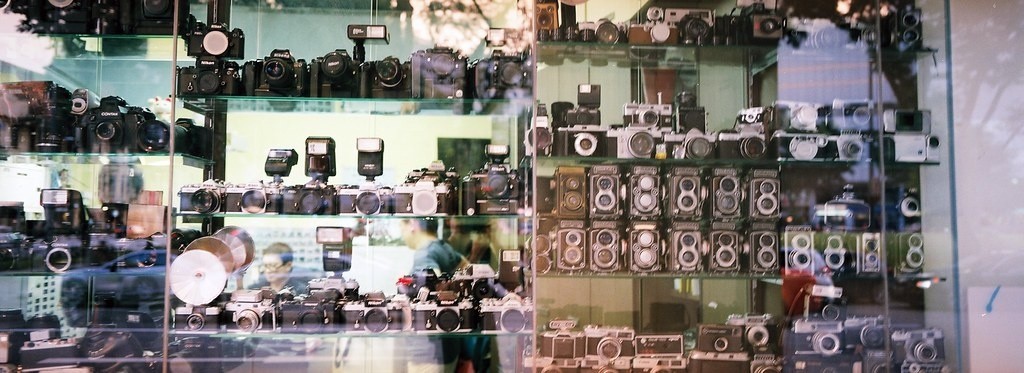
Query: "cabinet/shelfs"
0 0 963 372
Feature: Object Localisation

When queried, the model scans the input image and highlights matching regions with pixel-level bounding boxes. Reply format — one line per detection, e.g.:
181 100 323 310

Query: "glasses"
260 262 286 273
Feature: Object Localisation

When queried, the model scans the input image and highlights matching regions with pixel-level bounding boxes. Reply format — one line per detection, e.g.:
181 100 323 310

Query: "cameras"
0 0 949 373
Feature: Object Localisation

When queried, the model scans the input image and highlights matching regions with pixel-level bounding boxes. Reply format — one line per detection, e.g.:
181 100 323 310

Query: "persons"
404 216 504 373
245 241 324 373
